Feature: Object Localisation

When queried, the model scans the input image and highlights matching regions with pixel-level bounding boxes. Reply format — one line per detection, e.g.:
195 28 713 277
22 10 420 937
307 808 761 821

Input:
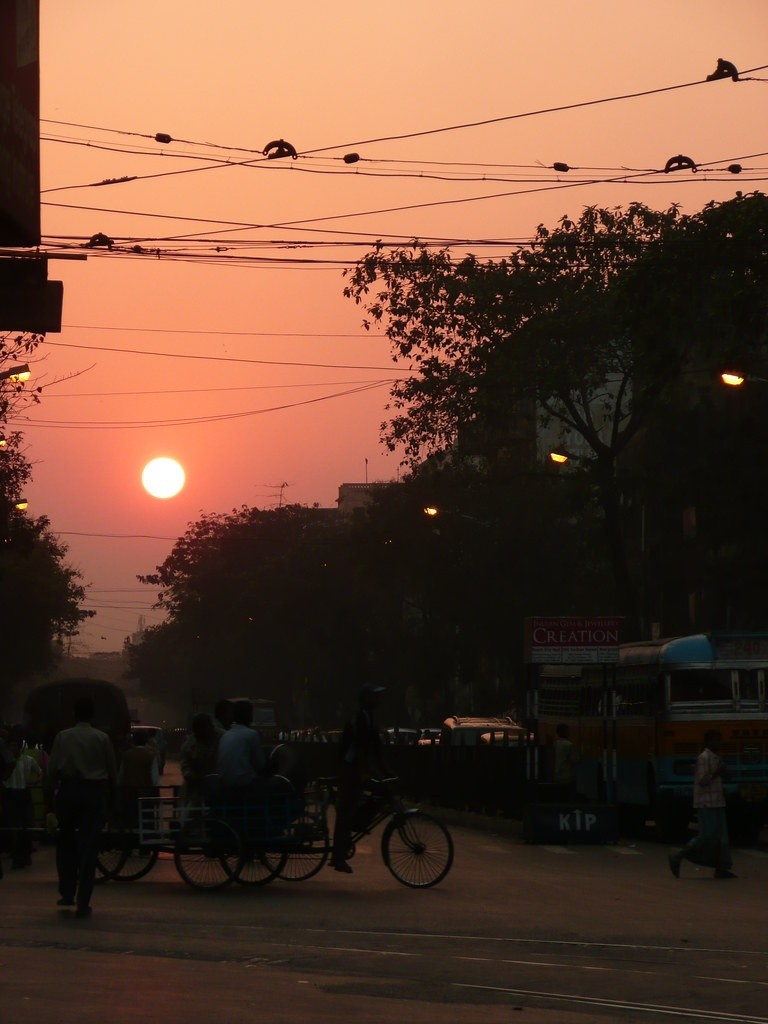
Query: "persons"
0 697 269 916
668 730 738 879
330 684 396 873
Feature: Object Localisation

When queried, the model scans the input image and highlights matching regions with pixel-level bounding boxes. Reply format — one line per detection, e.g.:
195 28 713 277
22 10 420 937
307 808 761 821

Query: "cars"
383 711 530 777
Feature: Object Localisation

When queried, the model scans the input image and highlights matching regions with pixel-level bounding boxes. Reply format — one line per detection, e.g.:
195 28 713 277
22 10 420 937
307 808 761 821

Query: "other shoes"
76 907 91 917
669 855 680 878
714 869 737 878
57 899 75 905
328 862 352 873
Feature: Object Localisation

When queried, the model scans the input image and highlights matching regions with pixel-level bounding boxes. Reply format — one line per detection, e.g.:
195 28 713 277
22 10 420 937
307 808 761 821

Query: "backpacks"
262 734 307 788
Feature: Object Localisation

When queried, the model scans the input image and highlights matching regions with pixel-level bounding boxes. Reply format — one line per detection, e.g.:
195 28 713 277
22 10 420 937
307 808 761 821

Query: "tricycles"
86 777 455 892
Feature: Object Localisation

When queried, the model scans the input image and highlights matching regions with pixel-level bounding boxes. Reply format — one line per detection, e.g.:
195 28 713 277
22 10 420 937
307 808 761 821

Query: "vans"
131 725 168 771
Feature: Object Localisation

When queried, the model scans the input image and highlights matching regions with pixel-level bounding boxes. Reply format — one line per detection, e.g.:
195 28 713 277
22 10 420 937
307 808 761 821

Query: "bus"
531 632 768 814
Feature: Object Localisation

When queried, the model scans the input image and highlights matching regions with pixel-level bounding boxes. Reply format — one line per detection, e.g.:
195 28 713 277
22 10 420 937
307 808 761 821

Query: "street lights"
543 450 651 630
